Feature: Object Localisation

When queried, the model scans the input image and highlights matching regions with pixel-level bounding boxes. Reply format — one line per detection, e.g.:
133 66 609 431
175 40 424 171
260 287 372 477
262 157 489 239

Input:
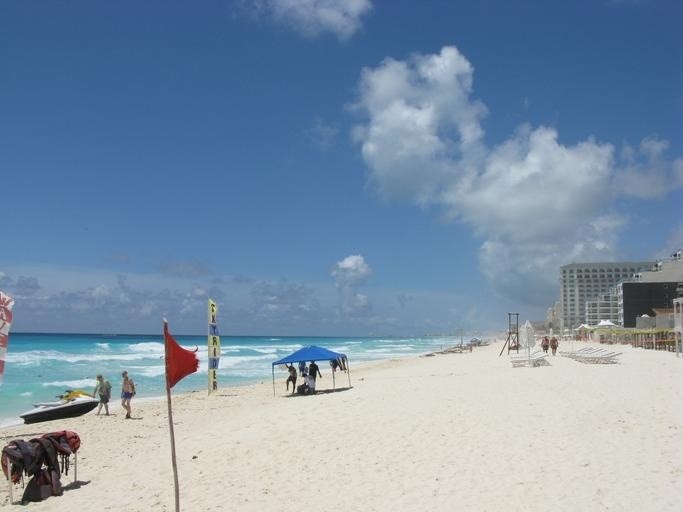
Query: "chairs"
510 347 622 365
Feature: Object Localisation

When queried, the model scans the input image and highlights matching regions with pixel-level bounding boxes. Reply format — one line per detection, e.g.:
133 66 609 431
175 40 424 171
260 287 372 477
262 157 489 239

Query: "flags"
167 331 199 387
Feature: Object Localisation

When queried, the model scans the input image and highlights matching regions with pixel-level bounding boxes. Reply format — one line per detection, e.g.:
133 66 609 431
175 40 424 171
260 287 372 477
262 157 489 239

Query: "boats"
19 388 101 424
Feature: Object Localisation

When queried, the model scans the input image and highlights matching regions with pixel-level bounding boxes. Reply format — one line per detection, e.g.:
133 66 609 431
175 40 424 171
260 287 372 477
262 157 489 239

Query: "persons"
120 370 136 418
303 373 315 394
309 360 322 382
550 336 559 354
93 375 112 415
298 361 306 376
541 336 549 353
286 365 297 394
